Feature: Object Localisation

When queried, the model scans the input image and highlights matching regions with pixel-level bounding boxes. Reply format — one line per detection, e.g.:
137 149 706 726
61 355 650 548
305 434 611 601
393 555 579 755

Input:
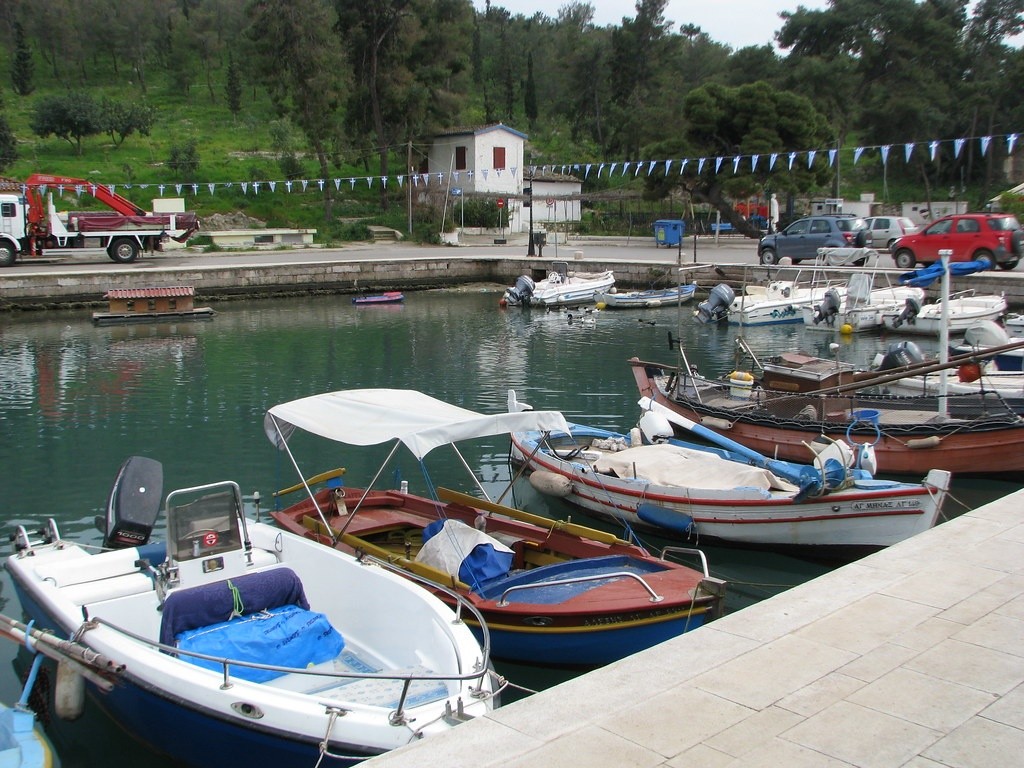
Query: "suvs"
757 213 875 267
887 211 1024 271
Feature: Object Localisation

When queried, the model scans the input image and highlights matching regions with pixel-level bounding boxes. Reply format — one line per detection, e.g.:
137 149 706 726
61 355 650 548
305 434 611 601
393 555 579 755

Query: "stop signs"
497 196 505 208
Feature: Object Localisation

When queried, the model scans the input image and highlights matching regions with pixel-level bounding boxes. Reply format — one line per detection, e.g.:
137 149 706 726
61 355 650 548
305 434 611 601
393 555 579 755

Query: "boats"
799 283 1010 335
593 281 697 309
624 356 1024 477
849 321 1024 414
4 480 502 758
91 285 219 327
691 248 856 326
497 262 618 307
351 292 405 303
506 389 952 548
263 387 729 667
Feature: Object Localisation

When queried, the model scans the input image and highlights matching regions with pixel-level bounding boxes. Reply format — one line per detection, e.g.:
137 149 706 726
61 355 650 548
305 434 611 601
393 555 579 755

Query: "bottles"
474 513 485 534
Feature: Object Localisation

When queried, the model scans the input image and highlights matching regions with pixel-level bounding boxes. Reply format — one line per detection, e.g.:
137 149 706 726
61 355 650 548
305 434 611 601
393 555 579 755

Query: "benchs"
158 568 346 685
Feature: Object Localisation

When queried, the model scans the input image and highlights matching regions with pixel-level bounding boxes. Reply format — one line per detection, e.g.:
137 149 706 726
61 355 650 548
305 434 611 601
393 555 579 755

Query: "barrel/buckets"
730 374 753 397
849 410 878 427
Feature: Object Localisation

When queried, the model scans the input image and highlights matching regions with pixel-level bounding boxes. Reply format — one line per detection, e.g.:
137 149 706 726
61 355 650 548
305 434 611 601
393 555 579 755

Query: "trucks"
0 173 200 266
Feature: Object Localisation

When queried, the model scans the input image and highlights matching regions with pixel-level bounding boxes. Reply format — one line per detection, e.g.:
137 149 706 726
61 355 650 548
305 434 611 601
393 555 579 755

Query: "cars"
859 215 920 252
